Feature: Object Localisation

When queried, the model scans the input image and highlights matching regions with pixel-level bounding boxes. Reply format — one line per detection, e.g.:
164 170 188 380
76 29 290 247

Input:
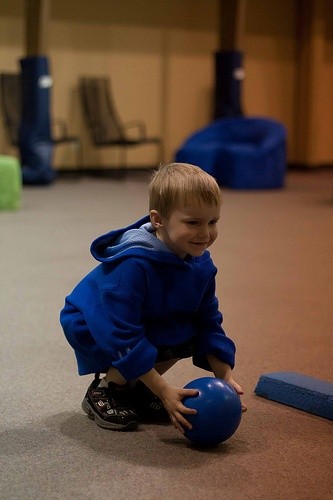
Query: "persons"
59 163 247 435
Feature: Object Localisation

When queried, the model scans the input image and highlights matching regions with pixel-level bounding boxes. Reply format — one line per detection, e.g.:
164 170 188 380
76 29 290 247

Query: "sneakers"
81 378 137 431
131 380 184 425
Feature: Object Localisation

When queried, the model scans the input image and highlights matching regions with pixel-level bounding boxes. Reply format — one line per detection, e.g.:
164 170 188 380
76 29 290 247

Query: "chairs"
79 74 166 181
0 69 100 177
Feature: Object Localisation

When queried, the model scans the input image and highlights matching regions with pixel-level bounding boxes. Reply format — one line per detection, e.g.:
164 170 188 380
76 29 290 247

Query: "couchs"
173 114 290 191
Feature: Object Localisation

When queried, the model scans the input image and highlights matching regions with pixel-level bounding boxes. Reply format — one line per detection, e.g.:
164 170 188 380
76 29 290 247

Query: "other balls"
177 377 244 448
